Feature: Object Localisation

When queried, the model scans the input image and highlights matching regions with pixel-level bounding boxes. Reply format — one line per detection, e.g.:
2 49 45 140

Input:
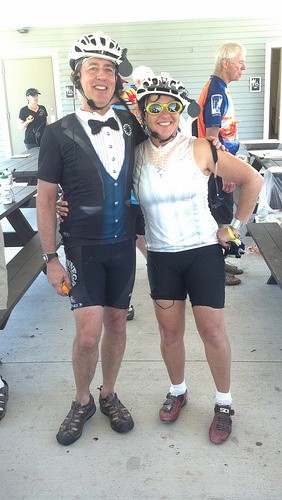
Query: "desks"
0 186 49 275
0 147 40 186
246 154 282 237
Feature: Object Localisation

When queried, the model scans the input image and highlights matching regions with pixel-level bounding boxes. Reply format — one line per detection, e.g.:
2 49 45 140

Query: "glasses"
143 101 184 115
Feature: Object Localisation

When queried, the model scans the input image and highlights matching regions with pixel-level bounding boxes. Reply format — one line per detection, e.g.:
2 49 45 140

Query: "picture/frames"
249 76 261 92
64 85 76 100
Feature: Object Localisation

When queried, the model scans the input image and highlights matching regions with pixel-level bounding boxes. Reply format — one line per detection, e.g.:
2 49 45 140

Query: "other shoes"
225 272 241 285
127 305 134 320
225 264 243 274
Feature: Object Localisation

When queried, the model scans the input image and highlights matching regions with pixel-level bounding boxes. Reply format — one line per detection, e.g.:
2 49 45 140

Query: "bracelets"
227 226 235 241
229 217 247 231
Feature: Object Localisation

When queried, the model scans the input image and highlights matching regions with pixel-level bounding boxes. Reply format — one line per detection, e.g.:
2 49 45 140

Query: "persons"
120 65 155 129
196 42 247 286
0 376 9 420
53 76 264 446
36 30 228 446
19 87 50 150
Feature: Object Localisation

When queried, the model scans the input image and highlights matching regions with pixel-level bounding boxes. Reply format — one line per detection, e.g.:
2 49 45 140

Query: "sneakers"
56 393 96 445
99 385 134 433
160 389 187 421
209 404 235 444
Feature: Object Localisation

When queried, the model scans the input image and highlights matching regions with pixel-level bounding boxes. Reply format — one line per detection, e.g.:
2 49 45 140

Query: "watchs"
42 253 58 263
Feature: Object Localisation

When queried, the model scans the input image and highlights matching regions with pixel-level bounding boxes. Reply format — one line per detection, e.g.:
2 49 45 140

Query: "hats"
26 89 41 96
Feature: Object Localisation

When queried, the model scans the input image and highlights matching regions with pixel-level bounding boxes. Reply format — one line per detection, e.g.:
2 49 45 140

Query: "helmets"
136 76 189 108
69 34 123 71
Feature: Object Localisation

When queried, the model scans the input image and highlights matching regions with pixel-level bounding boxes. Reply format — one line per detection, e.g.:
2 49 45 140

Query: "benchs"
0 231 61 329
246 222 282 290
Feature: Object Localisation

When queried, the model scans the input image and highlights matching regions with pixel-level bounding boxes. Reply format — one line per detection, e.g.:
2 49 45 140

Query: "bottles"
0 172 15 204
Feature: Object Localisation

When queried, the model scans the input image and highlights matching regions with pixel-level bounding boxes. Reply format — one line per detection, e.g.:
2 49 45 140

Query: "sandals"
0 379 8 419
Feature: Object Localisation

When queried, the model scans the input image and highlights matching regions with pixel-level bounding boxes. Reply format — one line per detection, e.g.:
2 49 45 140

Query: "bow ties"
88 117 119 134
